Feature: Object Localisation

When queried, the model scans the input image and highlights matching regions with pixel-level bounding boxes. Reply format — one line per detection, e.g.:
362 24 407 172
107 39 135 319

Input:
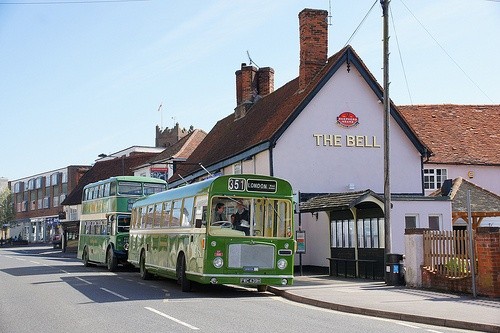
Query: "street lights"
98 154 126 175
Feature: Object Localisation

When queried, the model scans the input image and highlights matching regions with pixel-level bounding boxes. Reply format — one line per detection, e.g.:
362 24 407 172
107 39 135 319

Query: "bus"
126 173 297 295
76 175 169 272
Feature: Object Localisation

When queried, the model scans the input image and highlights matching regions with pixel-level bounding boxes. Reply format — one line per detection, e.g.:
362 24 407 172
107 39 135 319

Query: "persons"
235 200 254 236
86 218 129 234
212 203 229 226
231 214 237 229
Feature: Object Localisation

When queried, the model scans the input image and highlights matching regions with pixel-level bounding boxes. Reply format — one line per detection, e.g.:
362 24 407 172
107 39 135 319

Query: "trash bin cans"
53 240 61 249
385 254 405 285
